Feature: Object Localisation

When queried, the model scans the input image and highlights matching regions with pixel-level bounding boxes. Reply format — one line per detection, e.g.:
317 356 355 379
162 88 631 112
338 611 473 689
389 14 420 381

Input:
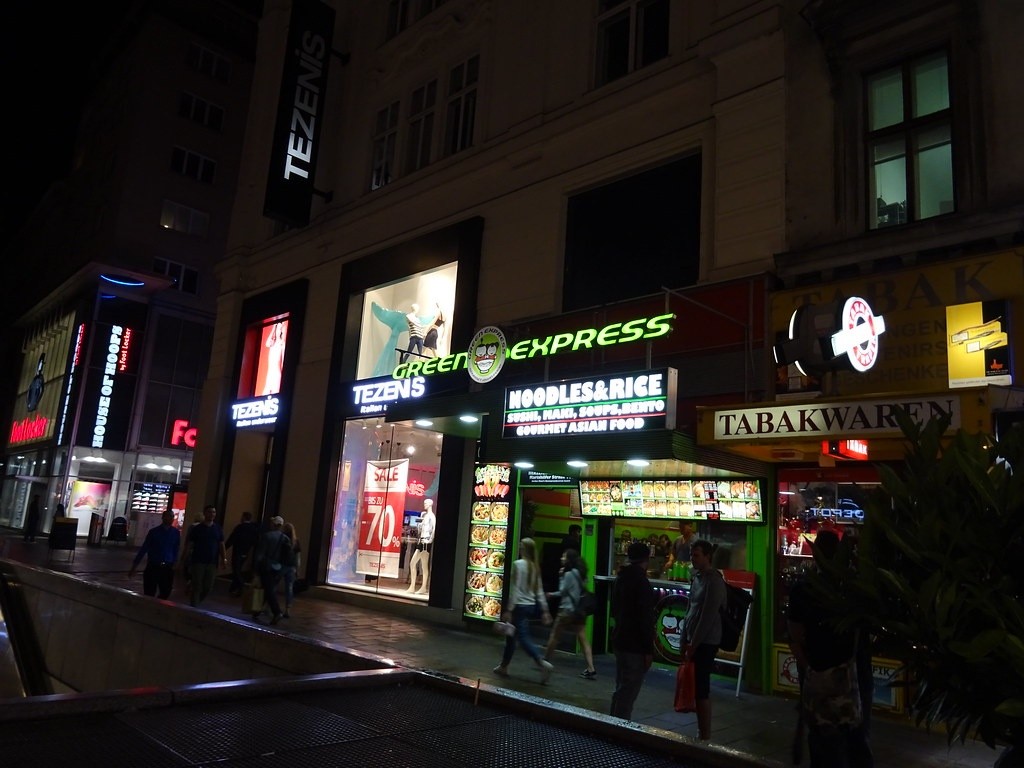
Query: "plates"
463 500 509 620
579 476 760 520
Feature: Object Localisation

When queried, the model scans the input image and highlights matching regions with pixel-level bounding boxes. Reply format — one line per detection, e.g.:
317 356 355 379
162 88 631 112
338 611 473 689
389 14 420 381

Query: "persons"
22 495 39 544
558 525 580 558
679 540 725 739
491 538 554 685
619 528 630 539
407 499 435 595
401 303 425 364
261 320 286 397
184 504 229 608
649 533 670 546
608 542 660 721
665 520 698 568
224 510 256 584
539 550 597 679
128 510 181 599
257 515 299 626
46 504 65 561
787 530 875 768
422 300 447 360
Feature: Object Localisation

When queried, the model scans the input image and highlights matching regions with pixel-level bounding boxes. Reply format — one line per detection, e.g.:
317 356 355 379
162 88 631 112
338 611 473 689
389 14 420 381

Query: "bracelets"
222 559 227 561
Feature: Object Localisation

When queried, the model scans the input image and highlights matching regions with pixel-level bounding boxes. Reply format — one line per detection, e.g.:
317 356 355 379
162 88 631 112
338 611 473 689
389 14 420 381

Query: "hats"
270 516 284 525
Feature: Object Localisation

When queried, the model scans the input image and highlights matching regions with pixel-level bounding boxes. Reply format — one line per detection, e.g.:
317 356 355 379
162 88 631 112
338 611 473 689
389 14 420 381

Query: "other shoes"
272 613 286 624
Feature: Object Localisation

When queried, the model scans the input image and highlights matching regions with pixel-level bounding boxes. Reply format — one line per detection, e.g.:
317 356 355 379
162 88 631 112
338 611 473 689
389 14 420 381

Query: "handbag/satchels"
493 622 515 637
240 577 264 612
675 643 696 713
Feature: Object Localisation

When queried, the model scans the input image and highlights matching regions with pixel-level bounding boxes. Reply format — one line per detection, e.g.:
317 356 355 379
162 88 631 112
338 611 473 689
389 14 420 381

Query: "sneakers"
580 668 598 679
494 665 509 675
538 661 552 685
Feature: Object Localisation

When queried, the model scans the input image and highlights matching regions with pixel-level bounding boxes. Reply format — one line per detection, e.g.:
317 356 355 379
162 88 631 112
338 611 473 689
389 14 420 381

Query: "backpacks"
561 569 601 619
712 570 752 652
